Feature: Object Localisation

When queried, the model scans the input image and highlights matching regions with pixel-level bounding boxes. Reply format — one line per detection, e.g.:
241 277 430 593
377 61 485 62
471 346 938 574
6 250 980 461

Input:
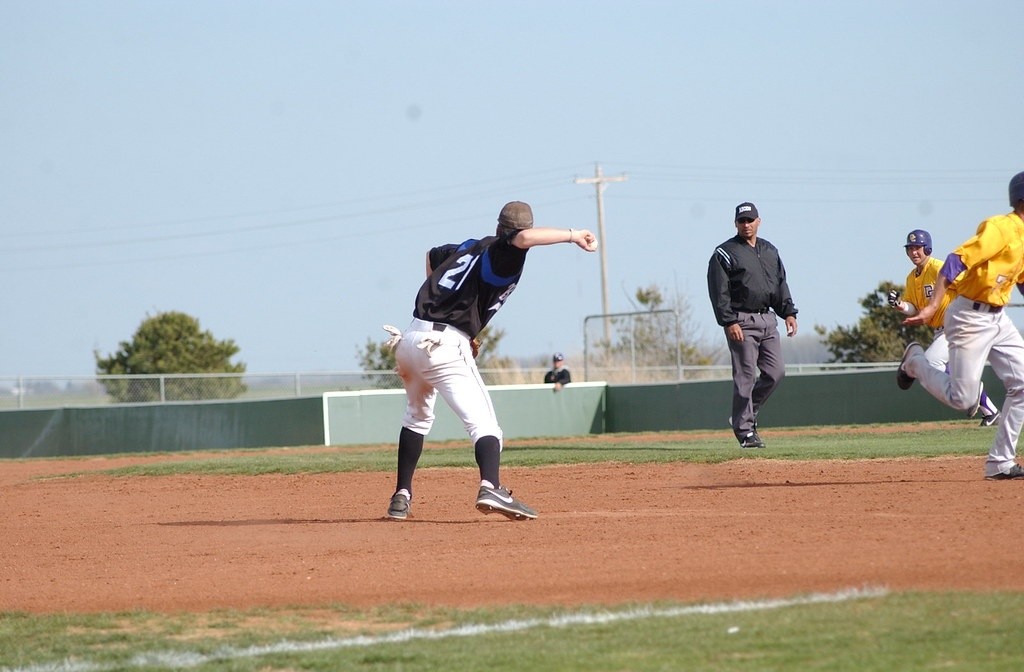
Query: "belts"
432 323 447 331
734 308 769 314
936 326 944 330
973 302 1002 312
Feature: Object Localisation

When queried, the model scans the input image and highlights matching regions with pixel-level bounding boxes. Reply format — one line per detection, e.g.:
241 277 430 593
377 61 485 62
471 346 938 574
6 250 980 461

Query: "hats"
553 354 562 361
735 203 759 220
498 201 534 229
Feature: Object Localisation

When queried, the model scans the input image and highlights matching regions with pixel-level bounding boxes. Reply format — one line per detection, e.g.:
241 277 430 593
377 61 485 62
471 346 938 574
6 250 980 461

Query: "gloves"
382 324 402 350
417 336 441 356
888 289 900 306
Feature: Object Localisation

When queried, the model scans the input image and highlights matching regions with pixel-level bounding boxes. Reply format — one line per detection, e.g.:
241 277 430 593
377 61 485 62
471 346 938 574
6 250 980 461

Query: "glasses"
737 217 758 224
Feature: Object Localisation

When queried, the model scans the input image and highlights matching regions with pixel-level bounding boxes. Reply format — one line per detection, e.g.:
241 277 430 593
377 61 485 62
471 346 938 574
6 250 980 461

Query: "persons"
707 202 798 448
545 354 571 393
893 230 1002 427
387 201 598 519
898 171 1024 480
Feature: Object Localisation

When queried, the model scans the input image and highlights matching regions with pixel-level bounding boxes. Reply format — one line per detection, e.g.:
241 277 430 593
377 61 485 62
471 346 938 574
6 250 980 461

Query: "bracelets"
570 229 572 244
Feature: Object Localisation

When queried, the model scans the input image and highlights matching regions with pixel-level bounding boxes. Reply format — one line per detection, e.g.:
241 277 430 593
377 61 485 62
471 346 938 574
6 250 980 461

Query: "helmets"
903 230 932 255
1009 171 1024 206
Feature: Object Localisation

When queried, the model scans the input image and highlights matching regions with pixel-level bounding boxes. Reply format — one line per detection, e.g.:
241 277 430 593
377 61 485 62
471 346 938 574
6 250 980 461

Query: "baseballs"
587 239 598 250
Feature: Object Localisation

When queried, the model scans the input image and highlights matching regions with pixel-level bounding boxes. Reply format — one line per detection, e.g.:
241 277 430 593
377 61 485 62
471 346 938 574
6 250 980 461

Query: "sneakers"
896 342 922 390
387 494 410 518
741 432 765 448
980 410 1002 426
985 463 1024 480
968 381 983 417
476 486 539 520
